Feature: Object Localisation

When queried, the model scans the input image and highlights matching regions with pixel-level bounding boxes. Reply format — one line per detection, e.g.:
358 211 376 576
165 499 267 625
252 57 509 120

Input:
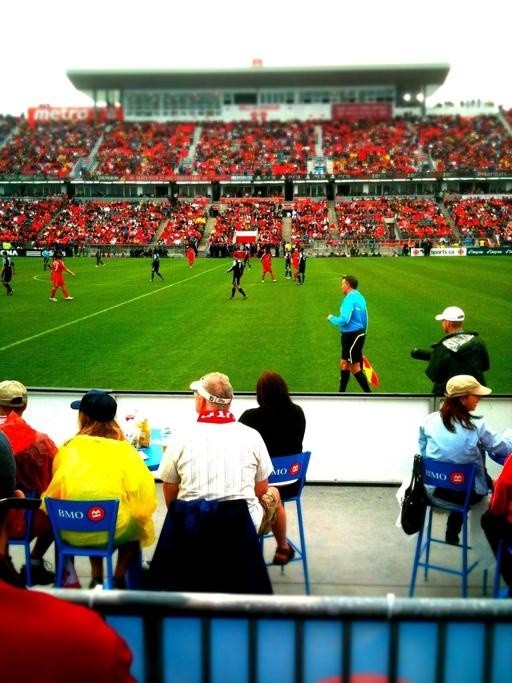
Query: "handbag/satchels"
401 454 426 535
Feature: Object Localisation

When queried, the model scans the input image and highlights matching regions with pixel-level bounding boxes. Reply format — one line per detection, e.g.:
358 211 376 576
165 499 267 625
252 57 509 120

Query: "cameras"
411 343 437 361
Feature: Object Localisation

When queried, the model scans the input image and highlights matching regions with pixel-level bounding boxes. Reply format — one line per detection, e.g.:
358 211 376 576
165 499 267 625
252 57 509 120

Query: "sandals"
273 543 295 565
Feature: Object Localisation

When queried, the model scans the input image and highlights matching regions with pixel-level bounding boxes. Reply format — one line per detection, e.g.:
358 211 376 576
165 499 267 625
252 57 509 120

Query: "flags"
361 356 379 387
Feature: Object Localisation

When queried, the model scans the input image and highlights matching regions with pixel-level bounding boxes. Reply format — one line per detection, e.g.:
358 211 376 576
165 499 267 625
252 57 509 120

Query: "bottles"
124 413 152 448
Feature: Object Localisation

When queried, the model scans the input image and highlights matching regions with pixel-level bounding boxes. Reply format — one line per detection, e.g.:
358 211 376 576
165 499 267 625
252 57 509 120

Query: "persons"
425 307 490 397
480 452 512 598
0 433 138 681
328 275 372 393
155 372 295 566
0 379 59 586
226 242 307 299
239 371 306 499
0 114 512 256
0 245 196 302
39 389 160 590
418 375 512 544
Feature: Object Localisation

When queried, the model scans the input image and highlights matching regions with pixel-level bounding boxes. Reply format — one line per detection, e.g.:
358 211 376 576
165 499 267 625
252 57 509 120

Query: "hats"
0 380 27 408
189 377 235 404
71 390 117 421
435 306 465 321
444 374 493 399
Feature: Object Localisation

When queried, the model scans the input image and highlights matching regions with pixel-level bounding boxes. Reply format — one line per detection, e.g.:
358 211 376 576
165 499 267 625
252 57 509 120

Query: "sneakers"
20 561 55 585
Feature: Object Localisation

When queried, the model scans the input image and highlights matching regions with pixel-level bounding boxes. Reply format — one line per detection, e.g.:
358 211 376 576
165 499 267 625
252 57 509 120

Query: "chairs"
262 452 312 595
45 496 132 589
8 490 36 586
491 544 512 598
409 456 487 599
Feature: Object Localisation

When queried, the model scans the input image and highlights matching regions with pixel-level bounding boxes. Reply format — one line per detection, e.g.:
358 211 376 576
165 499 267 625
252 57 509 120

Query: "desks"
135 427 164 471
